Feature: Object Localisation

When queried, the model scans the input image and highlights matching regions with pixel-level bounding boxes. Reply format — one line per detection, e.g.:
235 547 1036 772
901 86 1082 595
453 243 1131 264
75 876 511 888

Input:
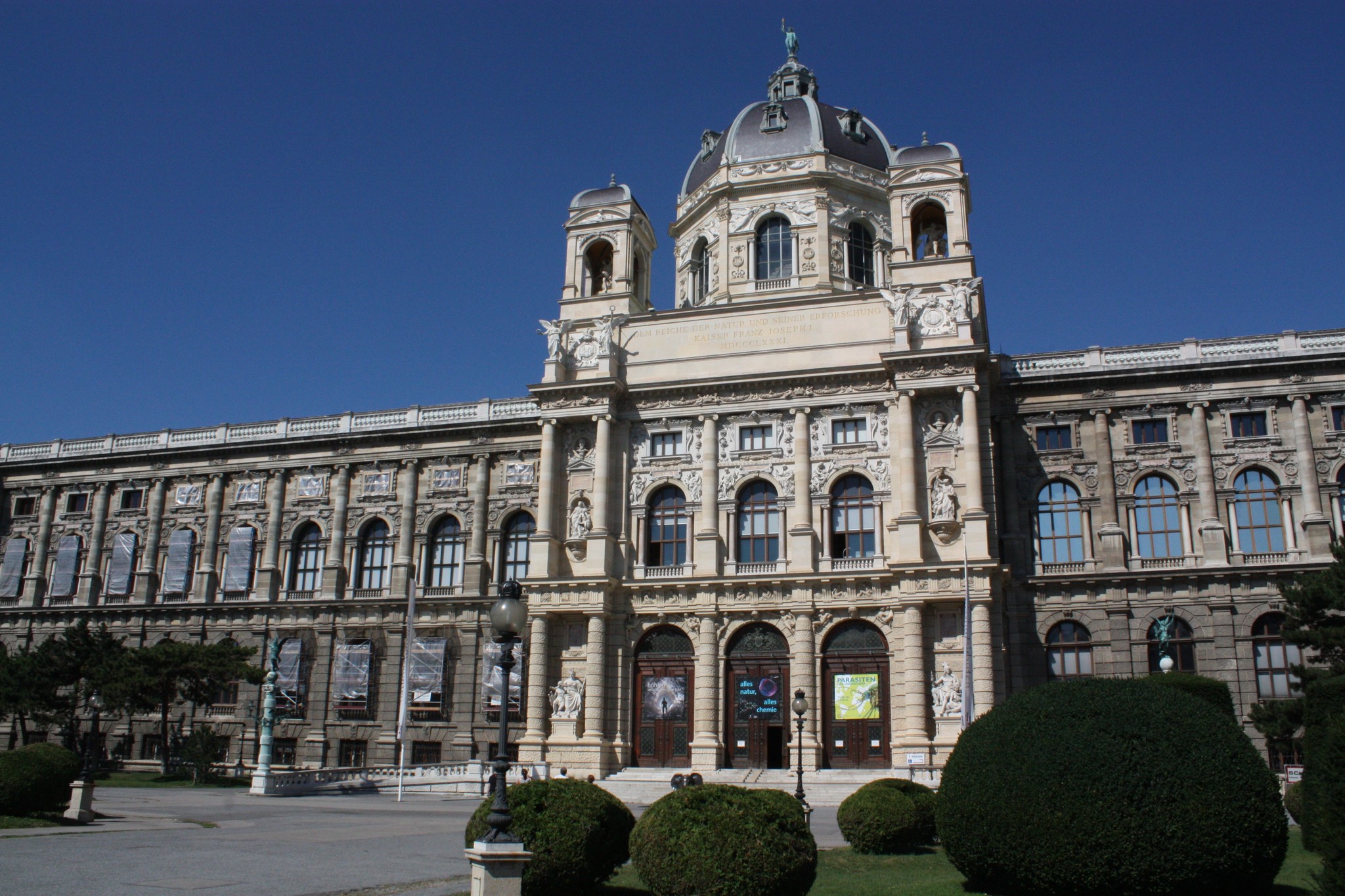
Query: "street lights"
248 636 288 773
62 689 105 822
791 688 814 825
464 577 535 896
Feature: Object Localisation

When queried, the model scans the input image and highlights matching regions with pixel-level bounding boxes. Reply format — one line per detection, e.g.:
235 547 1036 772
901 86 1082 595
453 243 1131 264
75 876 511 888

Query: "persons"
595 255 612 295
537 317 576 360
1149 612 1175 658
781 17 799 55
939 277 983 318
930 662 962 715
592 306 629 354
931 475 957 520
629 458 892 506
547 669 586 719
642 582 894 642
918 219 947 258
567 501 591 538
879 285 923 325
